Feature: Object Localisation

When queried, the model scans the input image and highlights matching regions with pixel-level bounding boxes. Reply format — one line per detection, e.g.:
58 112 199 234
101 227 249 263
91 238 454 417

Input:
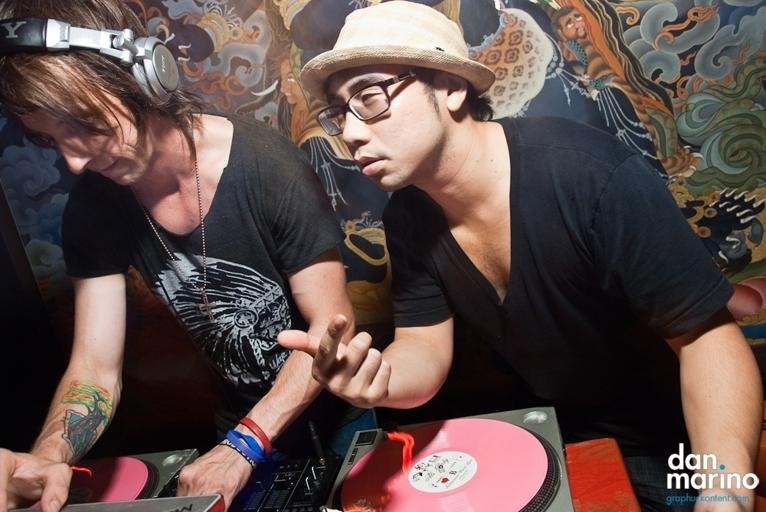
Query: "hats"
294 1 494 98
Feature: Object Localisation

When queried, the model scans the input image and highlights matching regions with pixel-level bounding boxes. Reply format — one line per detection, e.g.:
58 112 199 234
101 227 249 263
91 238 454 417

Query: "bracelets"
221 416 272 469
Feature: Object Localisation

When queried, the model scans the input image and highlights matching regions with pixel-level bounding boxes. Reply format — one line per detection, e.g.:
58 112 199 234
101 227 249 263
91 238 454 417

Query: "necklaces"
132 114 217 323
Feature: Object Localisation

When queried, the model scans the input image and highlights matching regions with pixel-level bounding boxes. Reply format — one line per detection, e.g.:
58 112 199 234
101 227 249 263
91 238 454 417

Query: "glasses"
311 65 432 139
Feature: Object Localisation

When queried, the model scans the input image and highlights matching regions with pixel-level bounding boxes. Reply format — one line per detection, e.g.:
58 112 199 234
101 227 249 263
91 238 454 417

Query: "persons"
0 0 382 511
275 2 763 511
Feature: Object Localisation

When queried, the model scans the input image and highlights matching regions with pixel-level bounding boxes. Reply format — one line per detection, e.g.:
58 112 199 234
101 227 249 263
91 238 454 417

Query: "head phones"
0 16 181 107
